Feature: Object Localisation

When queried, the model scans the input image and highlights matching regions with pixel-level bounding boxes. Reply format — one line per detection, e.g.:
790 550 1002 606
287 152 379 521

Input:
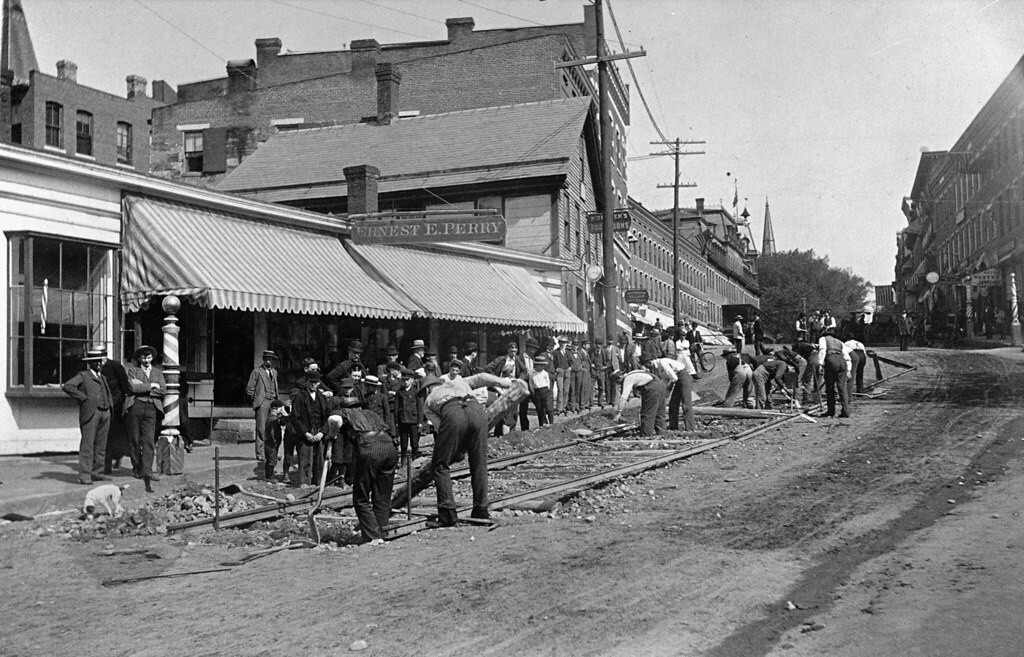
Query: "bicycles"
691 340 716 372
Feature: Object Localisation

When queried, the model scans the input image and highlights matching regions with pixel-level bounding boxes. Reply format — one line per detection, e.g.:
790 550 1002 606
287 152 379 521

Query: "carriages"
843 311 899 346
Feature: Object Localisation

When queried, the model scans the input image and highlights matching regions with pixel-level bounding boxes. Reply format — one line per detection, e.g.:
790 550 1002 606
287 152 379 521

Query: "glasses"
309 367 321 372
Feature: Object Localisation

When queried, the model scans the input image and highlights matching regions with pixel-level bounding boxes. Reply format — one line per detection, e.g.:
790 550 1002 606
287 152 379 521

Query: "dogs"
76 484 130 522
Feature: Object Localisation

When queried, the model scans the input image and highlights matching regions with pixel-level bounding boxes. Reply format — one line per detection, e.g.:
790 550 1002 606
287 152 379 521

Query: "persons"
246 310 873 544
885 302 1008 352
63 344 167 481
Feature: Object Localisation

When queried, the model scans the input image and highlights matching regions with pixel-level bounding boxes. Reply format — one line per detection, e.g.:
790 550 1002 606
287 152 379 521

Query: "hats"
337 398 364 408
257 350 278 359
463 342 478 351
82 346 108 361
524 320 699 382
305 371 321 382
302 358 317 367
385 346 399 355
341 378 354 388
502 364 514 377
901 310 907 314
348 340 364 353
133 345 158 360
720 310 854 374
361 375 382 386
449 346 458 353
418 376 442 397
411 340 427 349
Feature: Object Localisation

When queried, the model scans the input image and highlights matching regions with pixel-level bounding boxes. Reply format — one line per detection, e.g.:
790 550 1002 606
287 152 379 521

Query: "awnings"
121 194 590 334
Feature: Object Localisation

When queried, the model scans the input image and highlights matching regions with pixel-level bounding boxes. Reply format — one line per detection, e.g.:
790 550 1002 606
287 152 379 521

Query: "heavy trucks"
721 304 778 344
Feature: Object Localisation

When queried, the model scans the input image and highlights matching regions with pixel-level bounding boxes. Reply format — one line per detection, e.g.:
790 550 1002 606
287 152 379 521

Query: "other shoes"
470 508 491 520
838 414 849 417
438 507 458 527
821 410 835 416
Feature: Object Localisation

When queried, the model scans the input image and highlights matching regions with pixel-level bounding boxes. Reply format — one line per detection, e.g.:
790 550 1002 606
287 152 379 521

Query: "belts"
353 431 389 438
438 394 478 411
